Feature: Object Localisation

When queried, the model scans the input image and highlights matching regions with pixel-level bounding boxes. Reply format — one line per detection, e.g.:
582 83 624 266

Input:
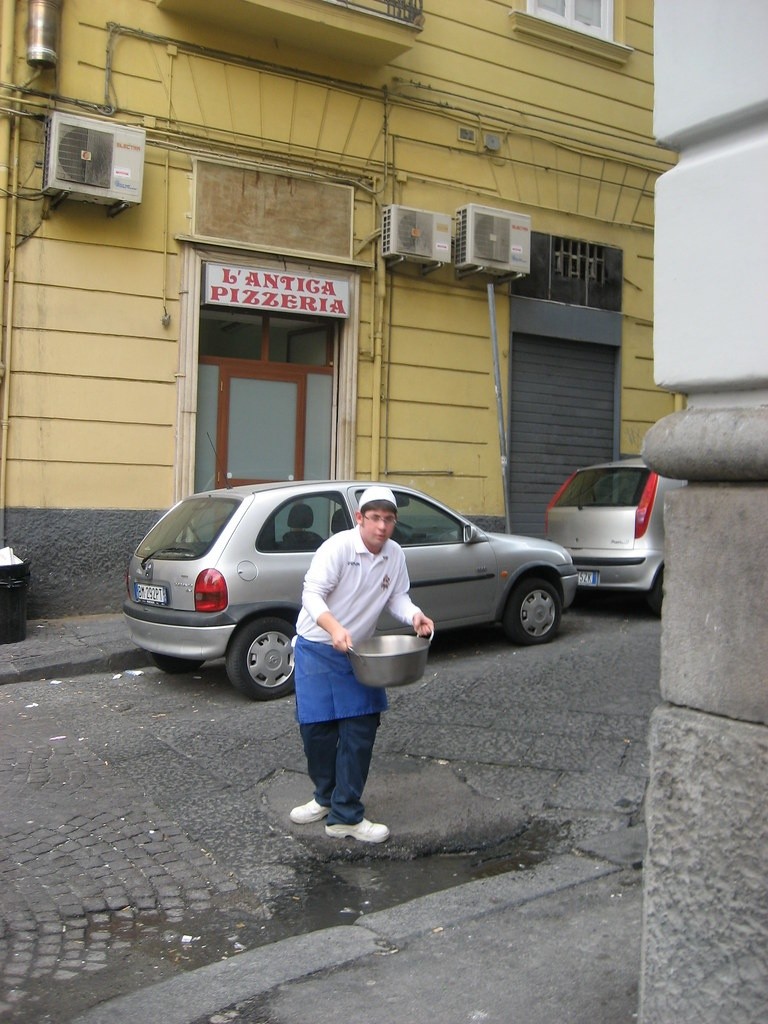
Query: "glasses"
364 515 396 527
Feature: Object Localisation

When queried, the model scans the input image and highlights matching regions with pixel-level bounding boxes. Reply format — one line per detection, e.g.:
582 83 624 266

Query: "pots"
345 623 435 689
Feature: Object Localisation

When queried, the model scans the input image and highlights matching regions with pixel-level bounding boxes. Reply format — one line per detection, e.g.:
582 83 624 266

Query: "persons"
290 486 434 843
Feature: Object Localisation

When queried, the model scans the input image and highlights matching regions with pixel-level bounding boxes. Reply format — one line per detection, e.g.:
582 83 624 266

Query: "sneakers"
290 798 332 824
325 817 389 843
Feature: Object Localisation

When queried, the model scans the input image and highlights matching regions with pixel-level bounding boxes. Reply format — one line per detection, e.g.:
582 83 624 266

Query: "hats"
359 486 397 511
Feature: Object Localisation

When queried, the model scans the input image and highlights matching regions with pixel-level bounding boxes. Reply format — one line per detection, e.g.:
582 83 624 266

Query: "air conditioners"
43 111 146 207
456 203 531 277
382 203 451 265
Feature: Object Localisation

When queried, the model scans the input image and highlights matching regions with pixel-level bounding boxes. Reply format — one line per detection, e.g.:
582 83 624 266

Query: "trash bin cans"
0 556 31 644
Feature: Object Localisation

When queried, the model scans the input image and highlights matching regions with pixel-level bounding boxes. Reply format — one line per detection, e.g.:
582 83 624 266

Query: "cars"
546 458 688 619
122 481 575 701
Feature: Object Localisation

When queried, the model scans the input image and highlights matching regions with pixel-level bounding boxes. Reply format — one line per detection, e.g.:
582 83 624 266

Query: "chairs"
331 508 349 534
282 504 325 550
